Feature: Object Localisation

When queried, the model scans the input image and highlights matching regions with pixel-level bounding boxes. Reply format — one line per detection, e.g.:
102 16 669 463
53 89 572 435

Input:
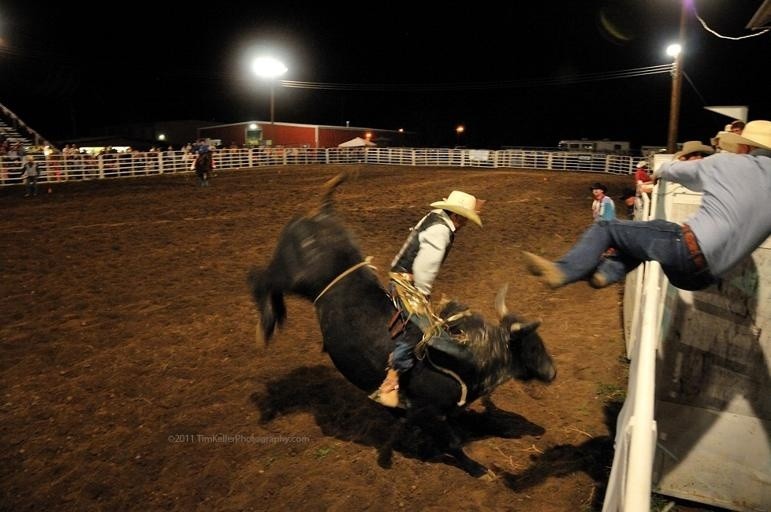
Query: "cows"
246 173 557 484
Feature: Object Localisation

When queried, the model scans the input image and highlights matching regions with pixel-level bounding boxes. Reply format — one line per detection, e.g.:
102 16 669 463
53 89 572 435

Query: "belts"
681 223 707 270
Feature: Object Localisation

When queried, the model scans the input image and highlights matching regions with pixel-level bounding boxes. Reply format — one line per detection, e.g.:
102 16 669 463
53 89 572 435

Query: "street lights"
250 55 289 128
665 41 686 153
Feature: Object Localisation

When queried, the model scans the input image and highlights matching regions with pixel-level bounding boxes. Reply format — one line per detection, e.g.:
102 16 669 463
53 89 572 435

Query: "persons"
591 181 618 261
388 189 489 373
668 119 746 163
519 119 771 291
623 184 637 222
0 129 365 189
634 158 656 189
20 154 41 197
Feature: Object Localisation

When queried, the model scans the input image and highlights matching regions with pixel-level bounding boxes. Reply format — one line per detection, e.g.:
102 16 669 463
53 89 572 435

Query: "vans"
555 137 671 169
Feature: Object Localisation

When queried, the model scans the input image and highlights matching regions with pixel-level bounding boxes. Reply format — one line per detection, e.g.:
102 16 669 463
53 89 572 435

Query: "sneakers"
520 250 566 289
580 272 608 288
378 368 399 408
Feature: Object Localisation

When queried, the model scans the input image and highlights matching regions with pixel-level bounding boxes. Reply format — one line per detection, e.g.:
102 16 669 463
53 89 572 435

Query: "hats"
429 191 483 229
672 141 715 160
636 161 646 168
711 120 771 153
589 183 608 193
619 188 635 200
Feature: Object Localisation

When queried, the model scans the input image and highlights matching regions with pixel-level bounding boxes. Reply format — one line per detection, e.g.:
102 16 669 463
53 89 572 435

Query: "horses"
196 153 210 188
